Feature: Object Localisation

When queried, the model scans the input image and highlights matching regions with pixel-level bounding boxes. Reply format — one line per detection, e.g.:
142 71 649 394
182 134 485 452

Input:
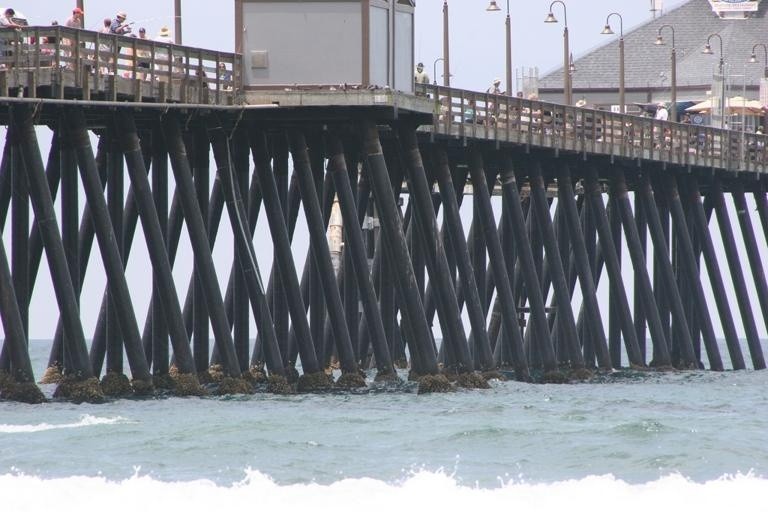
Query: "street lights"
653 24 678 122
544 1 570 104
432 57 453 86
484 0 513 97
569 53 578 106
601 13 625 113
701 33 725 128
746 43 768 79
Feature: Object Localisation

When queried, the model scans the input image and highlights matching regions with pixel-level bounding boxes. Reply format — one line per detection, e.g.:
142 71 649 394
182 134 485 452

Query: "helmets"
116 11 127 18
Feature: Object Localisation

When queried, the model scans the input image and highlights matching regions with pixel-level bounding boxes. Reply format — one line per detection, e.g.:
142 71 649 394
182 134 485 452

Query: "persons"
0 5 173 81
414 62 429 95
483 76 767 162
217 63 233 92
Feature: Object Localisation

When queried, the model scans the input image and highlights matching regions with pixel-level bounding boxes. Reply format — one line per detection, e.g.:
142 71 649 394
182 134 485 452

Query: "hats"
139 28 146 32
575 100 587 107
71 8 84 16
158 25 171 36
416 63 425 68
492 78 501 84
529 94 538 99
